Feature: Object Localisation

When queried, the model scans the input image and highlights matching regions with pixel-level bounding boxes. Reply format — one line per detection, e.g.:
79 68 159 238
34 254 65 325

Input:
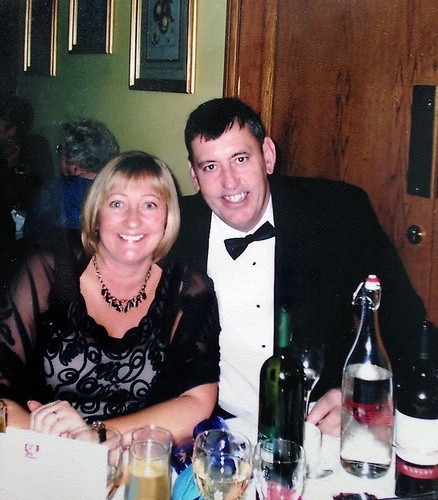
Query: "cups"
253 439 306 500
70 426 122 487
192 429 252 500
124 425 173 500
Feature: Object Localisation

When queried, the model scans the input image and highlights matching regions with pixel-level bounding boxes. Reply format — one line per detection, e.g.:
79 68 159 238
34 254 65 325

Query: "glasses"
55 144 69 158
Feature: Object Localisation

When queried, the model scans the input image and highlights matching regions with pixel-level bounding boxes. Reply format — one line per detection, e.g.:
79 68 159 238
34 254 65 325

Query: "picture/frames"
68 0 114 55
24 0 58 77
130 0 197 95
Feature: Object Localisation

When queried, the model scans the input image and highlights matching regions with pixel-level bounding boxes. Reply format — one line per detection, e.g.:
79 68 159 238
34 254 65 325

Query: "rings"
53 411 59 421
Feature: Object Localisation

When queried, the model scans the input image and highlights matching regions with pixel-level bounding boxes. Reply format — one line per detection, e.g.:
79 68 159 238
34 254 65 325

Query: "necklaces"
91 250 152 312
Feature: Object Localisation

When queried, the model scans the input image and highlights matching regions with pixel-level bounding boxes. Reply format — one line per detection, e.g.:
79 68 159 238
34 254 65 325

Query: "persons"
164 97 427 439
0 113 55 281
1 150 223 468
23 114 119 242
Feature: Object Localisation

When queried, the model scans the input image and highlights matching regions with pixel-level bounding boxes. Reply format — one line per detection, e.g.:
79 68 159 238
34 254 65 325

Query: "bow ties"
224 221 275 260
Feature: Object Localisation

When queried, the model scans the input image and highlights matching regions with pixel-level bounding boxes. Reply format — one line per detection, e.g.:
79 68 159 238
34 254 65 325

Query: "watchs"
91 420 109 445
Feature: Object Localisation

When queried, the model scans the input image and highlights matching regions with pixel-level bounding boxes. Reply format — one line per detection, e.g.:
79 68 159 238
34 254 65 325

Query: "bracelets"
107 427 121 439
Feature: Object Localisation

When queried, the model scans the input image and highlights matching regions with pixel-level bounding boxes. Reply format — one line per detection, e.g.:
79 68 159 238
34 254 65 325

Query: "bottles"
339 275 394 478
256 306 304 485
394 319 437 498
